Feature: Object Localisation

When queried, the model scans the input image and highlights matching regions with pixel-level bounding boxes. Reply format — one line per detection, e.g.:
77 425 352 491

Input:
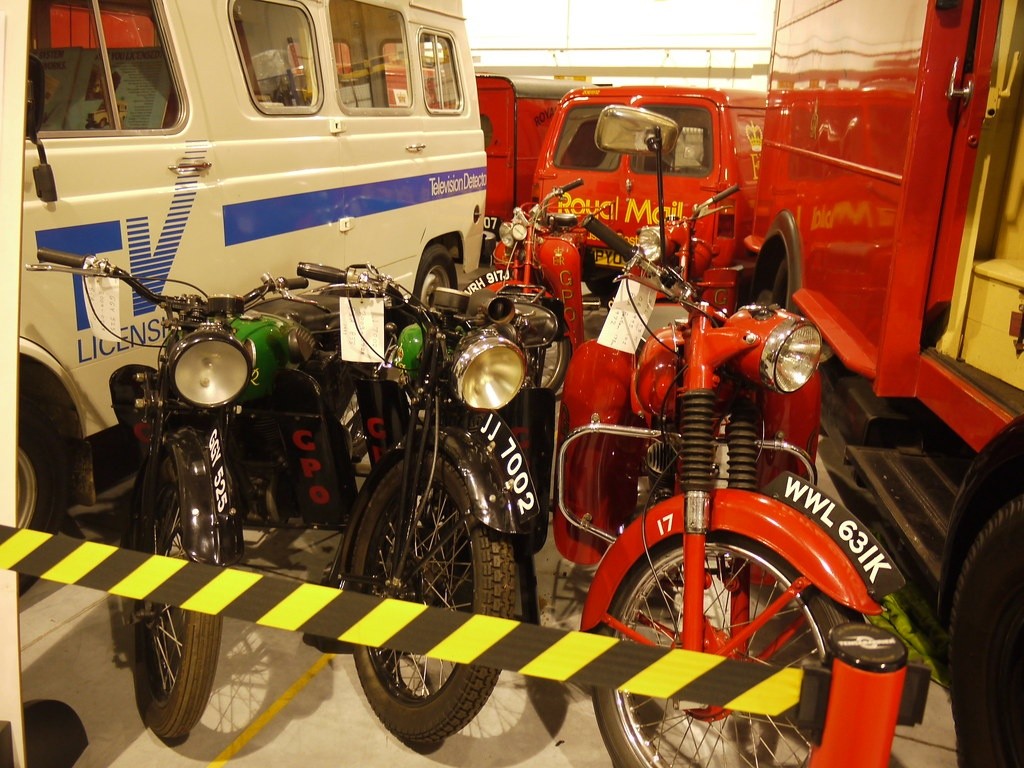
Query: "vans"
533 85 768 292
3 0 488 598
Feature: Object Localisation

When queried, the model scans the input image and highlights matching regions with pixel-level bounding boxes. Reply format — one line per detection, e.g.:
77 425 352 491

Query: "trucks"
744 0 1024 768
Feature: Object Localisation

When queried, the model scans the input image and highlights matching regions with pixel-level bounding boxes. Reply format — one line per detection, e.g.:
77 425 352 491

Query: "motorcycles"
27 246 411 739
550 183 907 767
297 175 601 747
